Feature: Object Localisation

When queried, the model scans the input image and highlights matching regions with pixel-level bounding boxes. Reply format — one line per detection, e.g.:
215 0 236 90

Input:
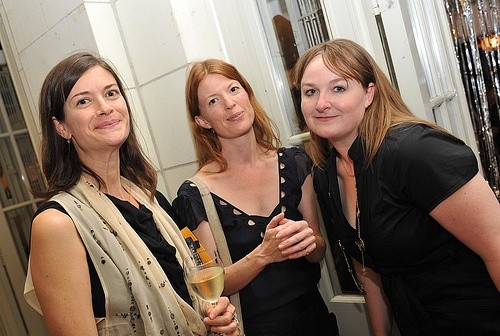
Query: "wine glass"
184 248 233 336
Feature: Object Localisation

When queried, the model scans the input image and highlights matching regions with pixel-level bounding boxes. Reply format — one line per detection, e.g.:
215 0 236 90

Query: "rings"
237 327 240 332
226 311 234 316
305 249 309 255
232 318 240 326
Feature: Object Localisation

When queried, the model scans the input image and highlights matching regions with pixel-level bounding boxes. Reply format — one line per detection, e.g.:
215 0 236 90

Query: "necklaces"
339 173 367 296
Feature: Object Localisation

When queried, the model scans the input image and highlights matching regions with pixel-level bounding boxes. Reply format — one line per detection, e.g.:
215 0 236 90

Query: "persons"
172 58 340 336
293 38 500 336
23 51 241 336
